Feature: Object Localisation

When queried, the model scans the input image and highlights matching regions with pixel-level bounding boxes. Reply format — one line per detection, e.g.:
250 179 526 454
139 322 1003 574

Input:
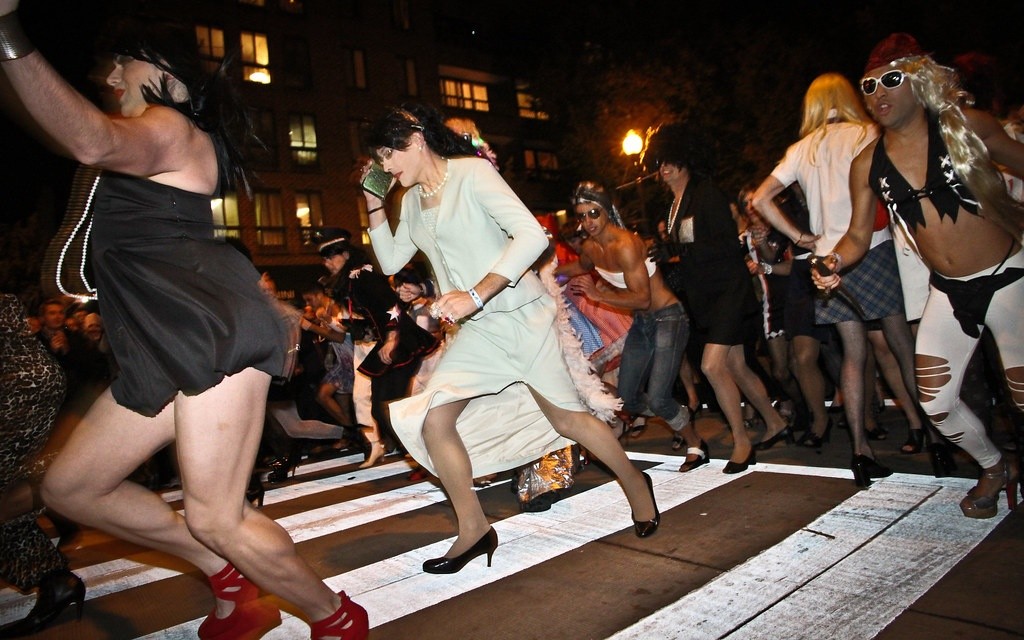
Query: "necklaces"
668 197 681 234
419 173 448 197
56 170 102 300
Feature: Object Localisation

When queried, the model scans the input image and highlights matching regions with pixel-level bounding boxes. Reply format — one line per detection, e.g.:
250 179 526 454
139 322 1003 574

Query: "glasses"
861 69 907 96
576 207 603 222
379 147 392 162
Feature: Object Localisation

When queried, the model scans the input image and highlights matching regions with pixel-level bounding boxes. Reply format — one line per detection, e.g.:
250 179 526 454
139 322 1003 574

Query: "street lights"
621 128 650 236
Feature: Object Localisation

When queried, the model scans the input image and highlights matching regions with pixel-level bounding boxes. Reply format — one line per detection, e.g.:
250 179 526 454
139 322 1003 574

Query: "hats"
645 123 700 174
571 181 612 222
862 32 931 78
311 226 351 255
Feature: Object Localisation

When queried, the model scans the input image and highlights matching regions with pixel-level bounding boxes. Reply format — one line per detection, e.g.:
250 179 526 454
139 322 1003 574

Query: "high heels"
632 472 660 537
959 456 1019 519
679 440 710 472
851 453 894 487
926 432 950 463
722 445 756 474
332 432 404 467
742 400 929 456
671 405 696 450
46 504 83 551
6 574 85 635
423 526 498 574
246 472 265 508
310 590 368 640
615 422 630 440
268 449 301 485
198 561 280 640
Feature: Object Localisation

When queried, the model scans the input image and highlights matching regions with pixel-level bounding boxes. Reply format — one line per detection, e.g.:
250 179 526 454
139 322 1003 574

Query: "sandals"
631 412 648 437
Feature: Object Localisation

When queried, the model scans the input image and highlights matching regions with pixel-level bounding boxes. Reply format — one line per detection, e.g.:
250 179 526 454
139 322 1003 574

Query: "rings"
444 314 455 324
428 302 443 319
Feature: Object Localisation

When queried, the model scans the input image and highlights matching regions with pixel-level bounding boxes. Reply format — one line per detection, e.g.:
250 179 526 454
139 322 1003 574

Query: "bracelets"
829 252 842 274
301 319 311 331
762 263 772 274
469 288 483 309
795 234 802 245
368 206 384 214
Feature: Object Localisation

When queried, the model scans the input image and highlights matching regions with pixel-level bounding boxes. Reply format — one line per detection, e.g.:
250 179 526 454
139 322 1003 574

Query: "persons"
0 293 85 640
360 100 659 575
445 32 1024 519
32 293 120 411
0 0 368 640
216 226 445 508
